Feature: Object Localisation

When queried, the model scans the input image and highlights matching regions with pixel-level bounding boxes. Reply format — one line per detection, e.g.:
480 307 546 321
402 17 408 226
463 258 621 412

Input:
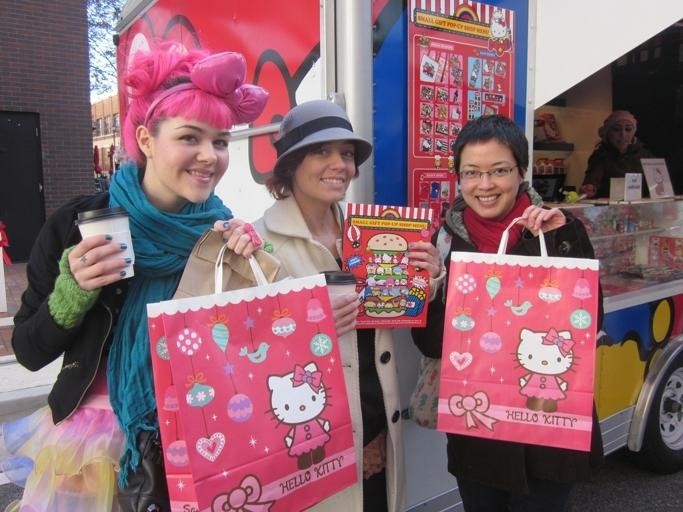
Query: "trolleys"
588 275 682 475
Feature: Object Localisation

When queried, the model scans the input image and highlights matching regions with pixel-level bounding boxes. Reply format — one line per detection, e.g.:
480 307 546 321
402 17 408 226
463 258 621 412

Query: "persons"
578 106 659 197
407 112 610 510
9 32 281 511
239 97 448 511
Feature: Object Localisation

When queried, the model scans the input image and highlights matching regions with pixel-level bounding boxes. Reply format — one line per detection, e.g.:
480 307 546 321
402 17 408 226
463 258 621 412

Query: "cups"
319 270 358 323
73 205 137 282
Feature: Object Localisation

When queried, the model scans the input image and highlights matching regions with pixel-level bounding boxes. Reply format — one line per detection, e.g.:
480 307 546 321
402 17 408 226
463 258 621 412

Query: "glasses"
455 163 520 184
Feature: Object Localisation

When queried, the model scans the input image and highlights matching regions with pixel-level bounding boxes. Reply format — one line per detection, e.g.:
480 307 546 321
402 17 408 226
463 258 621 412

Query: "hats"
599 110 637 143
274 99 373 183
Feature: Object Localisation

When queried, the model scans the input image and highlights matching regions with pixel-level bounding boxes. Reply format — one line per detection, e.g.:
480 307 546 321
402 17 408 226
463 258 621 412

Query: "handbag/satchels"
146 273 358 508
408 251 600 453
